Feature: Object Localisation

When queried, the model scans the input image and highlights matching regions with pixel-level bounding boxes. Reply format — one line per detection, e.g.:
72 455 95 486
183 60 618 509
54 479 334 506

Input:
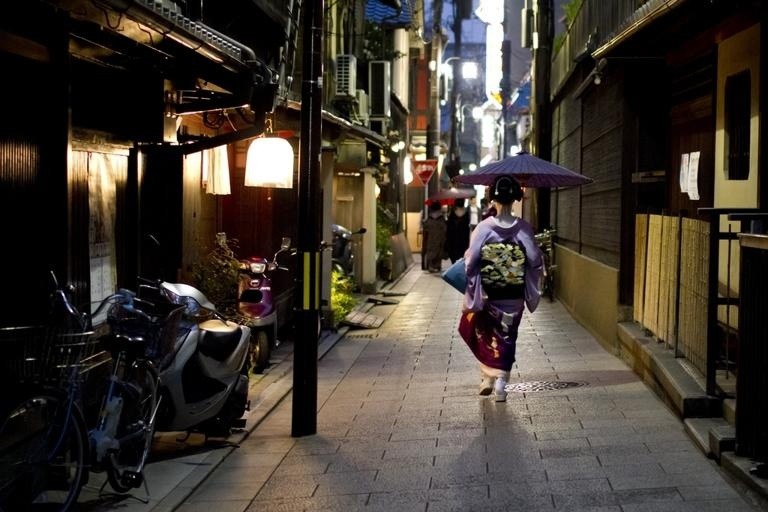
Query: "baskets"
22 332 96 390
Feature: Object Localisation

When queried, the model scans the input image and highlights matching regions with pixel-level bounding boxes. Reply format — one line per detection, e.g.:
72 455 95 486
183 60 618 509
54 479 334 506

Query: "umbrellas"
452 149 594 227
429 187 477 211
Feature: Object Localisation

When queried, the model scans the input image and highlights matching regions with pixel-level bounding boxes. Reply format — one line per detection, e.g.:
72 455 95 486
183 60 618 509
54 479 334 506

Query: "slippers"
495 392 506 401
479 384 491 395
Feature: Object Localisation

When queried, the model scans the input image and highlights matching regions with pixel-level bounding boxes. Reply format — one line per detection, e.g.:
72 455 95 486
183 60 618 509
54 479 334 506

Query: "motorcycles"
130 232 254 447
331 221 367 297
210 233 298 374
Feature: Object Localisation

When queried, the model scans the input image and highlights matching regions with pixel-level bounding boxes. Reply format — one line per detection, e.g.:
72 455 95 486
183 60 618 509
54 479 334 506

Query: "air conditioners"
336 53 393 136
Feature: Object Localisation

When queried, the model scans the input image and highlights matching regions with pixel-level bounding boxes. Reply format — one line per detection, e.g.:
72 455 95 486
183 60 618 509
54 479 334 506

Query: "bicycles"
1 252 190 511
531 227 562 302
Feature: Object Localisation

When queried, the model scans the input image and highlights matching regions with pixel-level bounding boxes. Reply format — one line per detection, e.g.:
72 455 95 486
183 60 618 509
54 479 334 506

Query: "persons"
419 195 491 275
457 175 543 403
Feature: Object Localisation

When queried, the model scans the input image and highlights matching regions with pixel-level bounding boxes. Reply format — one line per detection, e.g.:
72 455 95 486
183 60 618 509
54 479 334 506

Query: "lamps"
243 131 294 189
